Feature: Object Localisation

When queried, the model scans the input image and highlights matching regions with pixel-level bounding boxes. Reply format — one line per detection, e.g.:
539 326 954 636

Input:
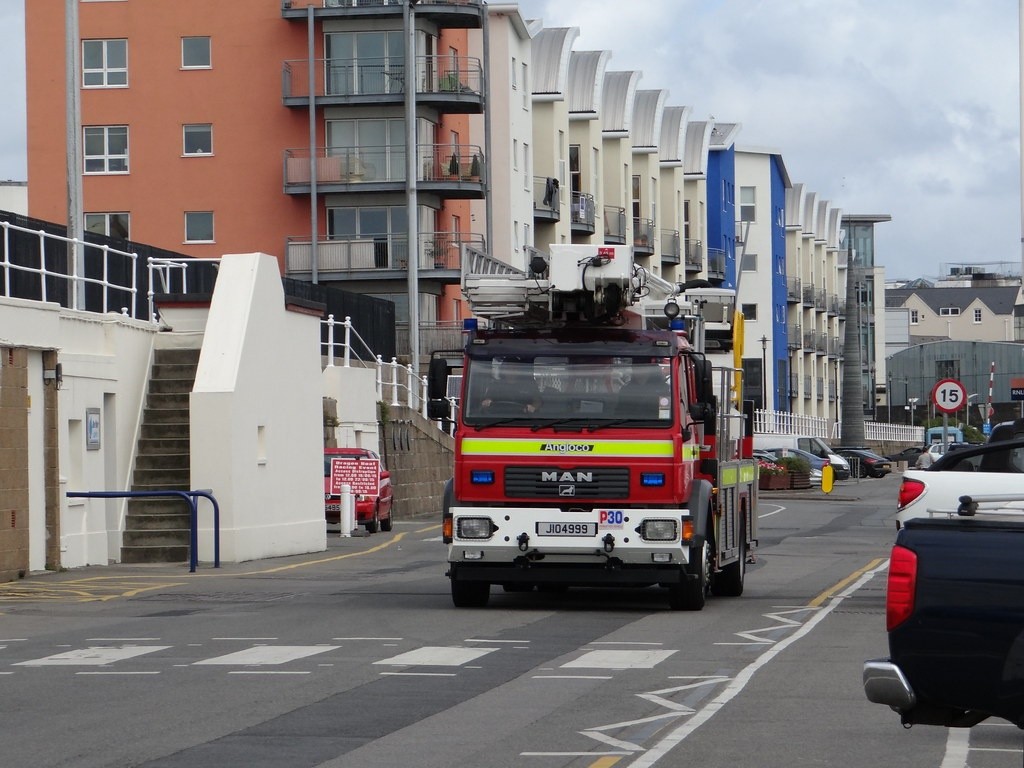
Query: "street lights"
888 371 892 423
870 366 877 422
966 393 979 426
904 377 908 425
757 334 771 432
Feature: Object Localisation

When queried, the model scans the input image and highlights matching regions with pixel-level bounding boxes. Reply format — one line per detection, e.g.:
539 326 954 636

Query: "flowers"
758 461 787 476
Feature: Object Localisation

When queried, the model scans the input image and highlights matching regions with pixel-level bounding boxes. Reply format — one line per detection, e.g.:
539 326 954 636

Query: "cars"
747 452 822 488
324 446 394 535
885 446 925 466
834 450 893 478
764 446 829 472
928 443 956 465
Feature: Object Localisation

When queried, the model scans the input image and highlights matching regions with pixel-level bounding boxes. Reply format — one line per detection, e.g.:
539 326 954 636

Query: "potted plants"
446 152 460 181
469 154 480 182
774 457 813 490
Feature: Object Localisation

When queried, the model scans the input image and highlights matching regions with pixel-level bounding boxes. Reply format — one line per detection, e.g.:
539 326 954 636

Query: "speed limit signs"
932 378 966 413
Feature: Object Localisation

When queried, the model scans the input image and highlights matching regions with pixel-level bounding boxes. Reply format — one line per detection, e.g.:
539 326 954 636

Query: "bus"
926 426 964 446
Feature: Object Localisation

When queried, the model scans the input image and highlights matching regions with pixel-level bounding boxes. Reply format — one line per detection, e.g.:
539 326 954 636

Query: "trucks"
751 434 851 484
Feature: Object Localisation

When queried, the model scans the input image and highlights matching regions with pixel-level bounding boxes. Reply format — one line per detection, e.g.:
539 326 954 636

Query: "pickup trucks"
895 438 1023 535
862 493 1023 735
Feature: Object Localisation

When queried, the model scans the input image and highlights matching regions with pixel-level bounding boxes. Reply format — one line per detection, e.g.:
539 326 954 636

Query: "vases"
758 470 789 490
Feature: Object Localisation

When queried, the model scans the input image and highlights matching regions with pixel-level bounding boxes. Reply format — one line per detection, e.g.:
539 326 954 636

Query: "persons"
613 355 672 420
478 355 544 417
915 447 936 470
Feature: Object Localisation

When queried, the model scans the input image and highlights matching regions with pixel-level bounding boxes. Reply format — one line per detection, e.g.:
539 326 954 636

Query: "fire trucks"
426 238 761 614
